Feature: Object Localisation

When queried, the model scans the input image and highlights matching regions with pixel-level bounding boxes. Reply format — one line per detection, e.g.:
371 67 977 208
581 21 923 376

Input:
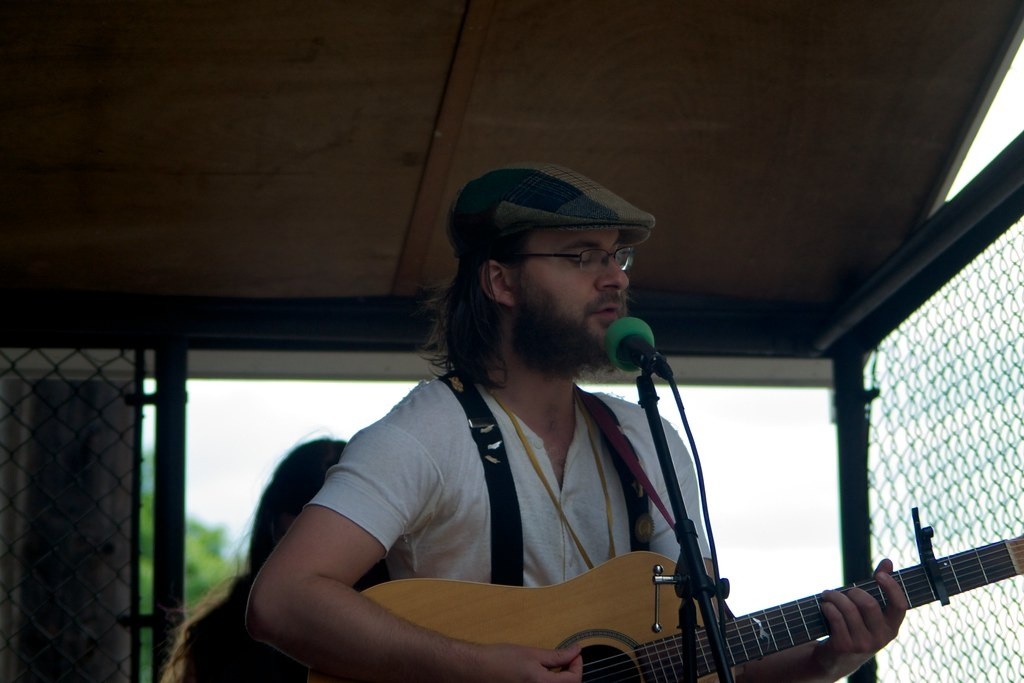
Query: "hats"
448 163 655 256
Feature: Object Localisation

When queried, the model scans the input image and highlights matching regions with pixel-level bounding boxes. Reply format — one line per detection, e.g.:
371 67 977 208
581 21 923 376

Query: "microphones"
603 315 674 380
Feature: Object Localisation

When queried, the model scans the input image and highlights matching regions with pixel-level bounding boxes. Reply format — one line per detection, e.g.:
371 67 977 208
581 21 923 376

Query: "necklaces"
479 379 616 570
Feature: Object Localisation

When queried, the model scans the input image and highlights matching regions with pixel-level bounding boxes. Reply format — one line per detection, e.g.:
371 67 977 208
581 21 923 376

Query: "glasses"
495 246 635 272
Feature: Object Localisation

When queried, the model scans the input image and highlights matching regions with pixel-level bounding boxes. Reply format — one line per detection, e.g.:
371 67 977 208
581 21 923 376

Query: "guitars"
304 533 1023 683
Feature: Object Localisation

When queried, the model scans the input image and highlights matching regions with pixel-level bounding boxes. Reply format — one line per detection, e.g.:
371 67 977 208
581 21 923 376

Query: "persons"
158 437 391 683
241 156 908 683
